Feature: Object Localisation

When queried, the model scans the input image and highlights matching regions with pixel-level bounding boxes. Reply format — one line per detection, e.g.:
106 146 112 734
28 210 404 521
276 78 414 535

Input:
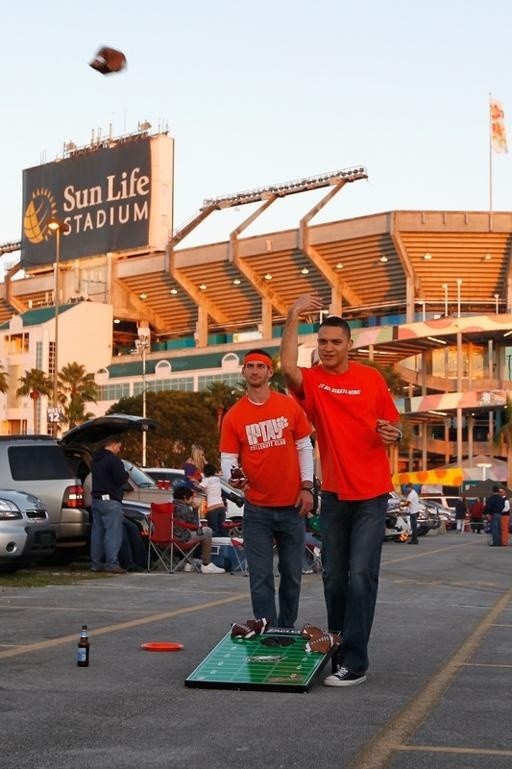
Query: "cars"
0 490 57 573
121 497 152 522
385 496 462 543
121 458 178 506
140 466 245 528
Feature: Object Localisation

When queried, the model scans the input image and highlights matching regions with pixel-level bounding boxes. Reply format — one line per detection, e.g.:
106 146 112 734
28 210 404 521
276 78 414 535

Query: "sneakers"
324 668 367 688
184 563 195 572
201 563 226 574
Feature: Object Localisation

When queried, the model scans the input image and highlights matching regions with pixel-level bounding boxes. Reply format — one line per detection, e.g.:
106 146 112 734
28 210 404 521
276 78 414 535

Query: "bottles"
75 623 91 666
304 511 323 533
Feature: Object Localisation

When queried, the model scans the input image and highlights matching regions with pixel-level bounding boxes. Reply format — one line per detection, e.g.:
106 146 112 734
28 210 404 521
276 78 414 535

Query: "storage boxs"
199 534 246 573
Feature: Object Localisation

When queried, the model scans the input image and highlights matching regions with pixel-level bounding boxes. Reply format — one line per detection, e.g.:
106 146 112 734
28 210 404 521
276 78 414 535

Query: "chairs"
219 507 243 536
302 525 323 575
231 534 279 576
143 502 204 573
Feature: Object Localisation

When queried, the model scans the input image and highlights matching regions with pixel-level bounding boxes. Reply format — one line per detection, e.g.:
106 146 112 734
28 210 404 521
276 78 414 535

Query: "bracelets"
398 429 404 441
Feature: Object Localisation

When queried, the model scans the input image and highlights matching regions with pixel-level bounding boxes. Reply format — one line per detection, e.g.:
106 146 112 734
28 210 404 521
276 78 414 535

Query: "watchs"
302 487 315 495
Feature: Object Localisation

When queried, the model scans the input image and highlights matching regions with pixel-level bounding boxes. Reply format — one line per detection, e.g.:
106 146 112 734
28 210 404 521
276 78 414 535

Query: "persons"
172 486 226 573
219 350 313 630
192 465 227 537
400 482 421 544
84 462 148 573
172 444 208 500
299 494 324 574
454 486 511 546
280 292 401 686
89 435 128 573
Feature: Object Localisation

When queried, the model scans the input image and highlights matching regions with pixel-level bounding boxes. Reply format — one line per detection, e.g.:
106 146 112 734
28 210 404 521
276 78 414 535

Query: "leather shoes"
104 566 127 574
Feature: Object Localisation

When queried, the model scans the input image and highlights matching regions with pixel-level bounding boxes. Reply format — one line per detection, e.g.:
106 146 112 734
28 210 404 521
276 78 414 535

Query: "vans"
419 496 481 513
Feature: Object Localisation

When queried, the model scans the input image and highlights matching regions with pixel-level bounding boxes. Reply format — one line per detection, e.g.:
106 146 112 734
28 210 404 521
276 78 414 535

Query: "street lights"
455 279 461 318
44 217 63 434
477 461 492 505
318 308 329 325
442 283 449 317
417 300 426 321
133 325 154 466
494 294 499 315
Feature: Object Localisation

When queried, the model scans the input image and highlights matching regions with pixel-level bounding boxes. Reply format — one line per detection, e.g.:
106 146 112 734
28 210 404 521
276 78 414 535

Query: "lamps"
204 166 366 207
379 256 389 263
170 288 178 294
199 283 208 291
232 278 241 285
483 253 493 262
335 261 344 270
264 272 273 280
424 254 433 261
301 267 309 275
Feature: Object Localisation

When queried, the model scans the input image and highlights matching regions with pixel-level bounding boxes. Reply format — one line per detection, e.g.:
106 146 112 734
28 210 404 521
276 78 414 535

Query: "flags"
489 95 509 155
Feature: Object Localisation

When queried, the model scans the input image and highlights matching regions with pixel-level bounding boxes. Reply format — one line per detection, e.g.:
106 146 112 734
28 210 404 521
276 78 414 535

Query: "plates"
141 641 186 652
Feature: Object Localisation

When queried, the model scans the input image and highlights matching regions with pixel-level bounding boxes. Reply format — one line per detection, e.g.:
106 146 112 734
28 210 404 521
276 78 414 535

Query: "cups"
163 479 171 490
157 479 163 489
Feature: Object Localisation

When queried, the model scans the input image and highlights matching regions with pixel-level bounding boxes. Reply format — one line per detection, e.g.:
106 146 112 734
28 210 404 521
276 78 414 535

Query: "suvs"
0 413 152 563
